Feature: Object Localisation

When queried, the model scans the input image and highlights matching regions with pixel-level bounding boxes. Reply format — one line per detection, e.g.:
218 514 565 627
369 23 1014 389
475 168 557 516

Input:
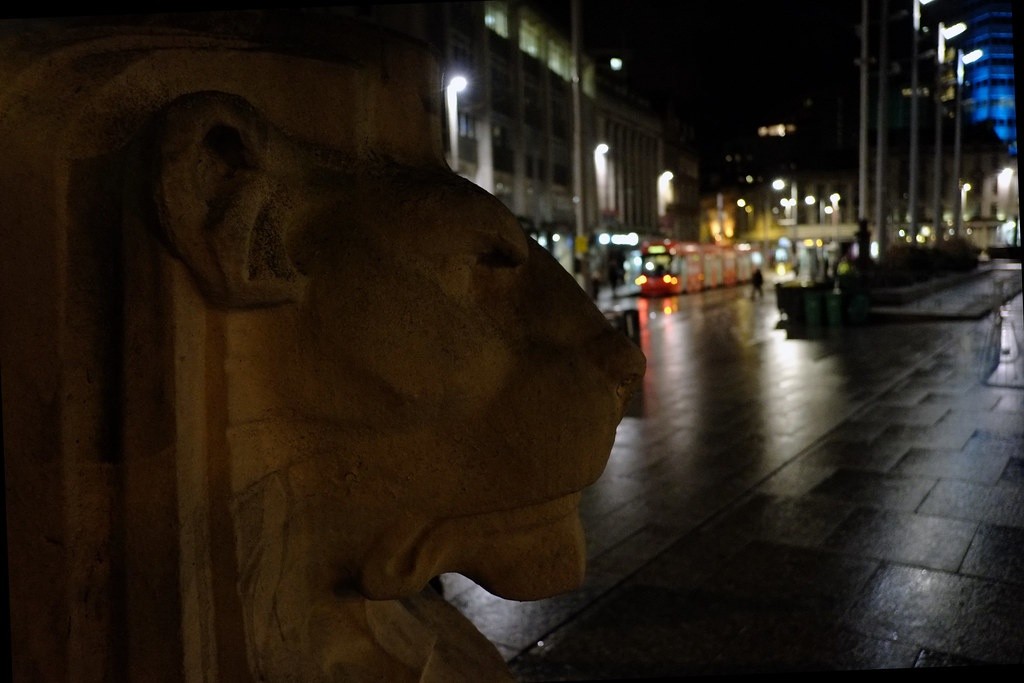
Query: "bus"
639 239 767 298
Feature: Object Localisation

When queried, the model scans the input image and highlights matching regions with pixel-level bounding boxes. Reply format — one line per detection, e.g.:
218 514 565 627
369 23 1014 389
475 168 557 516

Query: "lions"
0 14 643 683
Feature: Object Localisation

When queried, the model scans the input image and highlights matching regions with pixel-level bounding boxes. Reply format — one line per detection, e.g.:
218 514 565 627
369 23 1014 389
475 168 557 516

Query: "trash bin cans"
803 291 822 326
825 290 843 323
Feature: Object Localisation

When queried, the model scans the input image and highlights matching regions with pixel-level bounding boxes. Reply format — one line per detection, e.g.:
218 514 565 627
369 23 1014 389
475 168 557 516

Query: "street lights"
932 20 967 268
950 48 985 238
